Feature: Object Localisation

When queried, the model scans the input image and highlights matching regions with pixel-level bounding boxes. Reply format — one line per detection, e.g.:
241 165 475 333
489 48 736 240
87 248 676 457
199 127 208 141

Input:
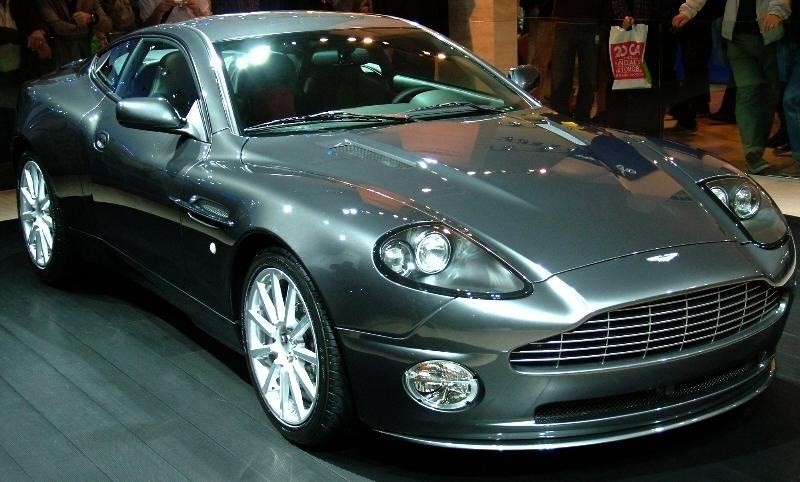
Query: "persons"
2 1 212 73
553 1 800 175
209 2 373 14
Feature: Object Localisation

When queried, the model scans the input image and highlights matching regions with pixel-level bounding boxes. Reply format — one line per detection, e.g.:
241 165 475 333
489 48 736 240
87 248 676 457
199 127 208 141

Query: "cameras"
174 0 187 8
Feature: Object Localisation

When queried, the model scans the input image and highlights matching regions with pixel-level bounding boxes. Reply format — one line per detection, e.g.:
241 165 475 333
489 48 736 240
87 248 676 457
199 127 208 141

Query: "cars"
10 10 798 451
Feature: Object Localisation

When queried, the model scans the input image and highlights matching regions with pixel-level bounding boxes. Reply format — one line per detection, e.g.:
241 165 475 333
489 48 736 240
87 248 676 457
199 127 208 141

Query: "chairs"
152 46 394 125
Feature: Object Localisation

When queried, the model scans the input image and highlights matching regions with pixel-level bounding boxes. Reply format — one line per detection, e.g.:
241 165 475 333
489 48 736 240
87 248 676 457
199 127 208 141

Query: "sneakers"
745 152 770 174
663 125 698 135
765 131 792 156
709 110 736 122
780 160 800 176
